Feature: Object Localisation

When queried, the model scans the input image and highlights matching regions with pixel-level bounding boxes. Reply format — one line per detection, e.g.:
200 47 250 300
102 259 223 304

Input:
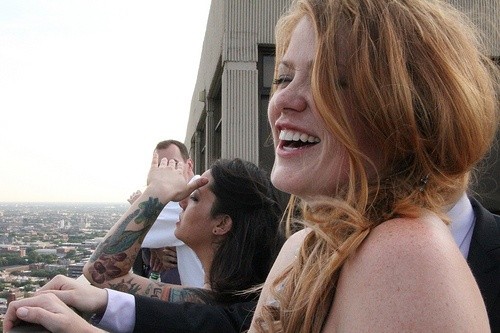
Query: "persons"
127 140 205 287
83 151 285 304
35 167 500 333
2 0 500 333
